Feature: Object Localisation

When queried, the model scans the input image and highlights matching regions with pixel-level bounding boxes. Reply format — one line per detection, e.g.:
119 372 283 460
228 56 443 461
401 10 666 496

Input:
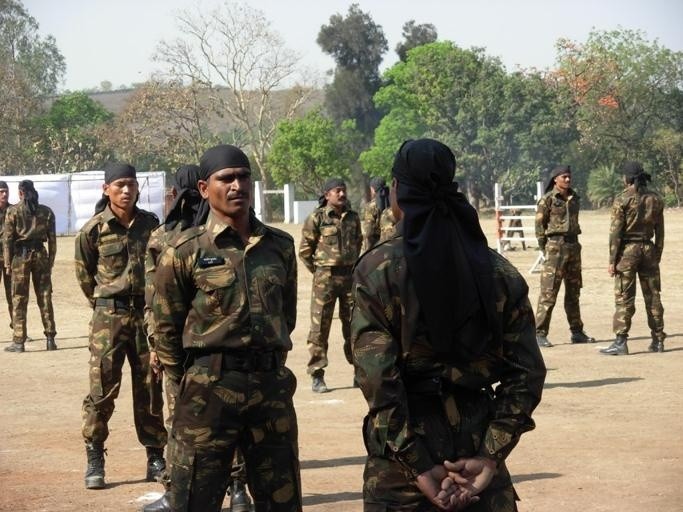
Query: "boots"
599 335 628 355
85 449 107 488
5 344 24 352
47 338 56 350
231 480 251 512
535 336 551 347
648 342 663 352
572 332 594 343
312 369 327 392
146 448 166 482
145 494 171 512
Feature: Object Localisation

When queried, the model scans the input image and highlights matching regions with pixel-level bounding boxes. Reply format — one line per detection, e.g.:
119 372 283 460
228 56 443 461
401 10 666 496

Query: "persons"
358 176 396 255
599 161 668 356
346 138 548 512
534 166 595 347
0 180 32 342
3 179 57 353
74 163 172 490
152 144 302 512
299 178 362 394
143 165 252 512
